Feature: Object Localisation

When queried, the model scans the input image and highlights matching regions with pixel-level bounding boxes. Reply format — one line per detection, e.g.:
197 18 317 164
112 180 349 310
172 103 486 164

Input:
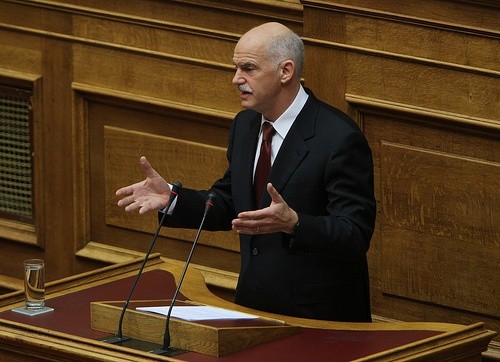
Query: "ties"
252 123 277 207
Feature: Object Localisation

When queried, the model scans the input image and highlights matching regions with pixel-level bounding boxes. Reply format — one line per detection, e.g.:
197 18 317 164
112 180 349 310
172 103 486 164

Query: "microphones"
149 190 217 353
100 180 182 342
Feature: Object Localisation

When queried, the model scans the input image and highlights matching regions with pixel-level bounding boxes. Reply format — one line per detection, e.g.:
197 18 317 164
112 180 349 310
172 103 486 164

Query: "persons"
116 22 377 324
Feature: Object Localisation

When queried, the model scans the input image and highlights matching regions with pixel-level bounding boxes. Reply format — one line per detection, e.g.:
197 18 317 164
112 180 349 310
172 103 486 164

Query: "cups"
23 258 45 312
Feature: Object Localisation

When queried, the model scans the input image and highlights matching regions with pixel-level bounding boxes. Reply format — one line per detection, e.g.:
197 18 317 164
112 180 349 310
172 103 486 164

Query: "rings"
256 227 261 233
